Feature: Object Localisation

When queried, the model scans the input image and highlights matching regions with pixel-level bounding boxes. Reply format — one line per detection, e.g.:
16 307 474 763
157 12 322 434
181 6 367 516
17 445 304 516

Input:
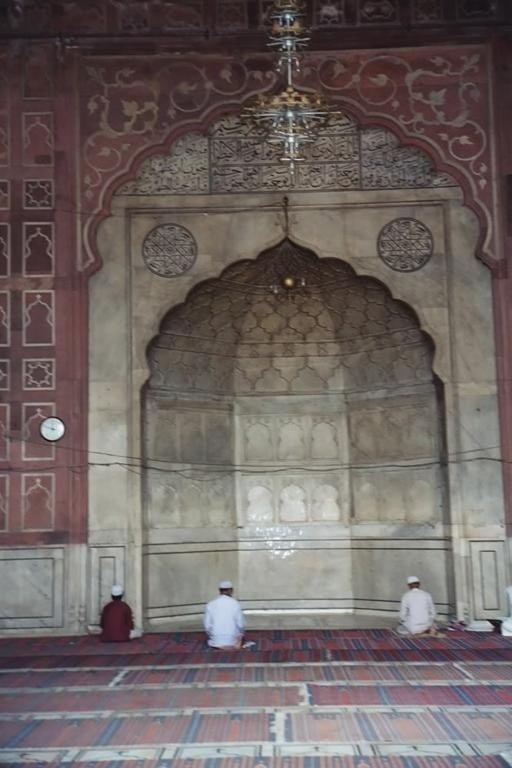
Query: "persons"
100 585 133 641
398 576 439 636
204 582 245 650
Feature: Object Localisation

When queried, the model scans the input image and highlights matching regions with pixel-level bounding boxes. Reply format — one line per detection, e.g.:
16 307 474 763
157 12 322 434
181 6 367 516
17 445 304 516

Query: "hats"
407 576 419 584
219 580 233 590
111 585 123 597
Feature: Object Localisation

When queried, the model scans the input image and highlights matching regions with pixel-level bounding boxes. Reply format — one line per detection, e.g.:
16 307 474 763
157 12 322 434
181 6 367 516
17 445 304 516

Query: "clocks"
39 415 67 442
